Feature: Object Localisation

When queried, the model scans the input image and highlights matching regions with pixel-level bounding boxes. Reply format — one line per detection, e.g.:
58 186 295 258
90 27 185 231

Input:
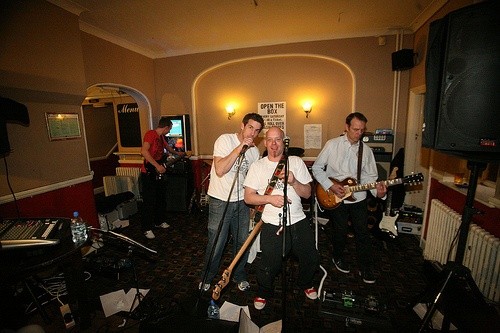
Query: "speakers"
392 49 413 71
420 8 500 165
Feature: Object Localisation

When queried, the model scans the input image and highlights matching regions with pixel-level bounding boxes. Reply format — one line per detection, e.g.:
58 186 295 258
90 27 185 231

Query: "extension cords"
60 302 77 329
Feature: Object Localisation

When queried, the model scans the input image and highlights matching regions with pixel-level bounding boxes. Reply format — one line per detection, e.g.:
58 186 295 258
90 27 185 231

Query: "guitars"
212 219 264 299
145 150 191 182
316 171 424 209
377 166 399 241
247 151 287 263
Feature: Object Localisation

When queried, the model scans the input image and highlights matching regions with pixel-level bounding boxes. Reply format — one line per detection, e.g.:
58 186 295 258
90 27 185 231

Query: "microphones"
282 135 291 152
240 138 253 155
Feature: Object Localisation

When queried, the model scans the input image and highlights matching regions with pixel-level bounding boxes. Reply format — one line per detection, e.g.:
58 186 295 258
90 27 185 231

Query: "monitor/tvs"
162 119 183 137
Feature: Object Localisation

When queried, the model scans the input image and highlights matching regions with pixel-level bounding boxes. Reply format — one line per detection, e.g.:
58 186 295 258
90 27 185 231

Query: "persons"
312 112 387 284
244 123 319 310
138 116 187 240
200 112 262 289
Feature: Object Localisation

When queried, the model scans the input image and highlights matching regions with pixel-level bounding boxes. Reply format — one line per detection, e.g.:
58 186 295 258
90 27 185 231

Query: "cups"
455 169 465 185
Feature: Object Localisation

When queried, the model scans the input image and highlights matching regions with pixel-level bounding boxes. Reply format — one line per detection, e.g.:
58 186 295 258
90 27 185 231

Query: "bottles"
208 300 220 319
71 211 87 243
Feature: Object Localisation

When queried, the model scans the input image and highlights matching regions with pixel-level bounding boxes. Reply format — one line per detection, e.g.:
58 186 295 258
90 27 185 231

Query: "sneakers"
358 269 376 283
253 296 265 309
143 229 155 239
199 282 211 291
331 257 350 272
232 278 250 291
155 222 170 228
305 287 317 299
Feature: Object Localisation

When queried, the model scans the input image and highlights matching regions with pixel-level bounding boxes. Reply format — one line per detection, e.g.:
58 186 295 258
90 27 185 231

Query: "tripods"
116 246 163 327
393 161 499 333
